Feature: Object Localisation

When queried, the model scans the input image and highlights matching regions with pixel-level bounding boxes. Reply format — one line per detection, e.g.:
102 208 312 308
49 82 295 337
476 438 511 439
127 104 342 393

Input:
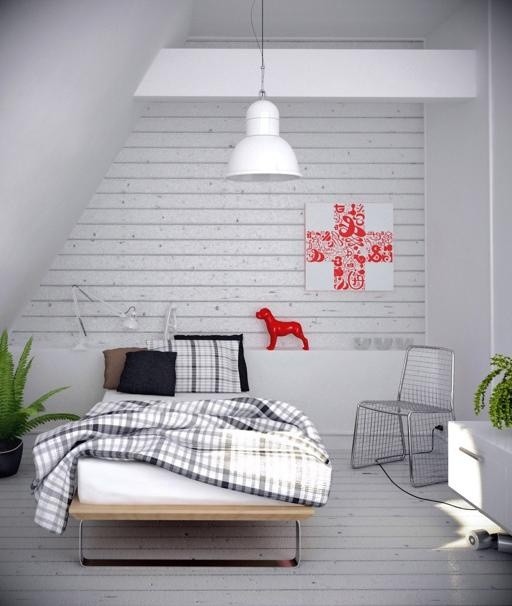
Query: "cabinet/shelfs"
446 422 512 534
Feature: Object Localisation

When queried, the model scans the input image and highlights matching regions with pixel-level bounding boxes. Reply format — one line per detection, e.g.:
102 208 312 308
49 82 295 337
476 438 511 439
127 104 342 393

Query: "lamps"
69 283 138 349
225 0 302 179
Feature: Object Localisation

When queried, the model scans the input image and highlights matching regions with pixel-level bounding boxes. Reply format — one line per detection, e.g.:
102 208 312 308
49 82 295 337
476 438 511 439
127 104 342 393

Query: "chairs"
349 343 456 488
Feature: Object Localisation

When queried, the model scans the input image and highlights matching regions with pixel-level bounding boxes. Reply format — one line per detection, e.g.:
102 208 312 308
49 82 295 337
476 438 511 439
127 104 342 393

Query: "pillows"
102 334 250 396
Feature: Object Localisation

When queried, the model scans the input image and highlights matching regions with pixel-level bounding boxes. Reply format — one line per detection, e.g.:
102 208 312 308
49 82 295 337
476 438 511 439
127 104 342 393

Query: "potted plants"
0 331 80 477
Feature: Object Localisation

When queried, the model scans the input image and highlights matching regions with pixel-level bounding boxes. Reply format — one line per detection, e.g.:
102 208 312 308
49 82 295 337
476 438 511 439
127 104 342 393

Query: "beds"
68 386 316 570
